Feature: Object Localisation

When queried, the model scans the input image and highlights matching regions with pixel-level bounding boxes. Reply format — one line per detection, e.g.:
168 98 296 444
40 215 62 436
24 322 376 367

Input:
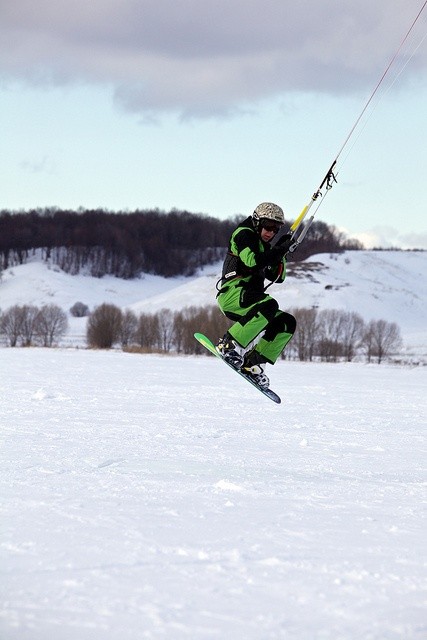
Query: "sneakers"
240 350 268 389
215 335 243 369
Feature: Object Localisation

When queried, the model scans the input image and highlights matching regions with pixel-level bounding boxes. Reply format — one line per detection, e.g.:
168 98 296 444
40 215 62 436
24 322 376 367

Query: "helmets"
252 202 284 225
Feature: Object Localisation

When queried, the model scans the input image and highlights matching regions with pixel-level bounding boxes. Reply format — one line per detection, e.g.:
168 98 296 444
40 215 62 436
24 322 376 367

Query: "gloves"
273 234 292 246
272 239 294 256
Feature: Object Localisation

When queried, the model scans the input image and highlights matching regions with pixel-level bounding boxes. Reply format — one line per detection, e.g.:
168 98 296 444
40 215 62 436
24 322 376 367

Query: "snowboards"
194 333 282 405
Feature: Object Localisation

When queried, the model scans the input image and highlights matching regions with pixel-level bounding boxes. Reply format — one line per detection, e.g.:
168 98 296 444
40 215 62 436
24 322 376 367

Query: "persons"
215 203 297 389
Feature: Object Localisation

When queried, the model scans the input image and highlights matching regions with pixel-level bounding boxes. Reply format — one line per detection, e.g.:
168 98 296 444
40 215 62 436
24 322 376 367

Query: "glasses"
261 221 279 233
